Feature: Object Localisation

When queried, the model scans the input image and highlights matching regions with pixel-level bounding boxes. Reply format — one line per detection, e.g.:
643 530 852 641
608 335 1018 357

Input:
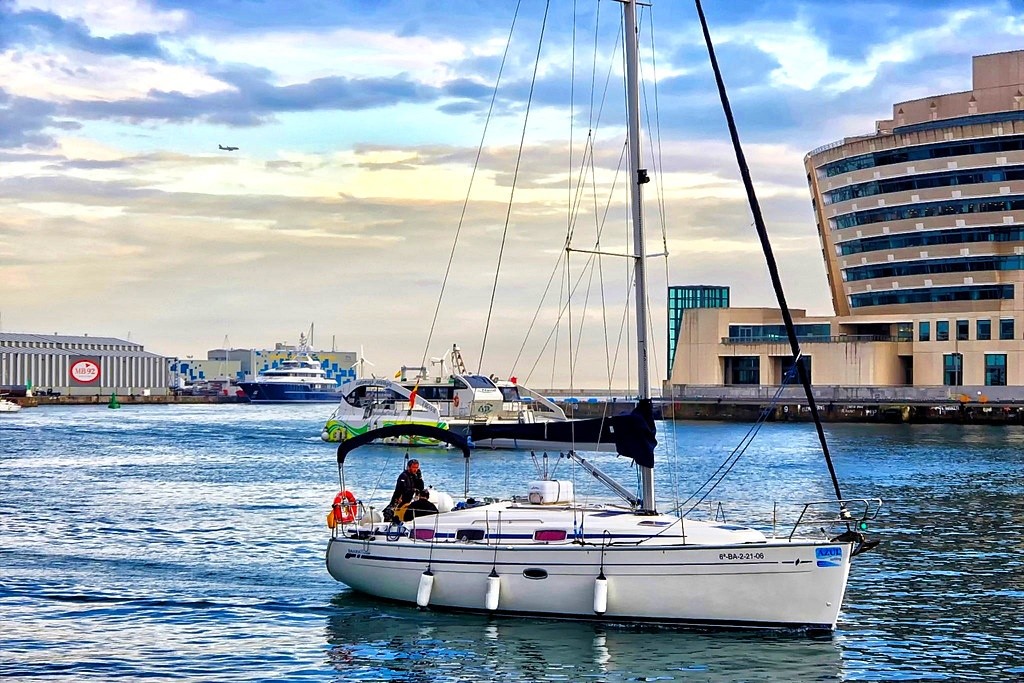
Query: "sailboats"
326 1 883 634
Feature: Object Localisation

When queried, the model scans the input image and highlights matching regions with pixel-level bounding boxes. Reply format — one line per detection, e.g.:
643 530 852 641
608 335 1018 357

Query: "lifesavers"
333 490 357 525
453 396 459 407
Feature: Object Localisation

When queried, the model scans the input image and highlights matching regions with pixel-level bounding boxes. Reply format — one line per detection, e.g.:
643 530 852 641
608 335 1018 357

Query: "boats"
236 333 386 403
0 399 21 414
321 344 616 453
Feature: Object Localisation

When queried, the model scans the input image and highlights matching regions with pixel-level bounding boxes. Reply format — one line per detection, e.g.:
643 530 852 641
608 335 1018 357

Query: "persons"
395 459 424 497
403 489 440 522
382 490 421 522
